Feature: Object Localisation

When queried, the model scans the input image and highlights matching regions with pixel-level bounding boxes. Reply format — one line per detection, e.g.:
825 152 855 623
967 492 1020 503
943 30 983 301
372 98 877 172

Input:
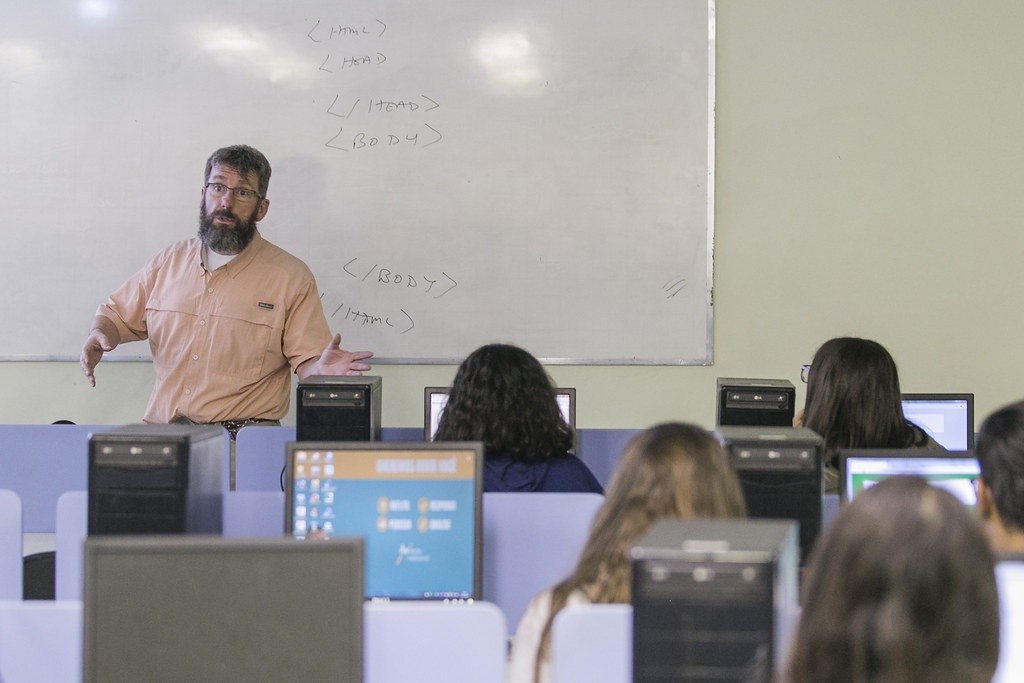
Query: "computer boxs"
713 426 825 565
629 515 800 682
86 425 227 532
716 377 795 425
297 375 382 440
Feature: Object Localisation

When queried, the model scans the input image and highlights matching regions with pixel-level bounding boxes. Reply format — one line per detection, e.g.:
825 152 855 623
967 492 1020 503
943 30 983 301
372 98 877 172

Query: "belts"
174 417 281 442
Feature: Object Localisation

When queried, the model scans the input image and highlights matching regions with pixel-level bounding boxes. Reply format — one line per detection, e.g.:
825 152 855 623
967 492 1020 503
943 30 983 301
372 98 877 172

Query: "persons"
428 343 604 493
790 336 946 495
784 473 999 682
975 398 1023 637
510 420 746 683
78 143 374 491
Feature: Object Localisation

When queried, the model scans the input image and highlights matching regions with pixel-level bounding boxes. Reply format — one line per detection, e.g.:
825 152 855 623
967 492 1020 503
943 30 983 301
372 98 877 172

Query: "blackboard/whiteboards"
2 1 719 365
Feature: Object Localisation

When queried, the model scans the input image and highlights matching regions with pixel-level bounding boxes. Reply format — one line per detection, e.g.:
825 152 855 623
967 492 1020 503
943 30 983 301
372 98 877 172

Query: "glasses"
205 183 262 202
970 477 980 498
800 364 812 383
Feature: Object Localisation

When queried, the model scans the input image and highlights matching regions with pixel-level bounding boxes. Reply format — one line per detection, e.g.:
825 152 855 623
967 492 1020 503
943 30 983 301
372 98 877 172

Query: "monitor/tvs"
839 447 982 516
423 387 577 457
900 392 975 457
80 533 366 682
283 440 485 602
